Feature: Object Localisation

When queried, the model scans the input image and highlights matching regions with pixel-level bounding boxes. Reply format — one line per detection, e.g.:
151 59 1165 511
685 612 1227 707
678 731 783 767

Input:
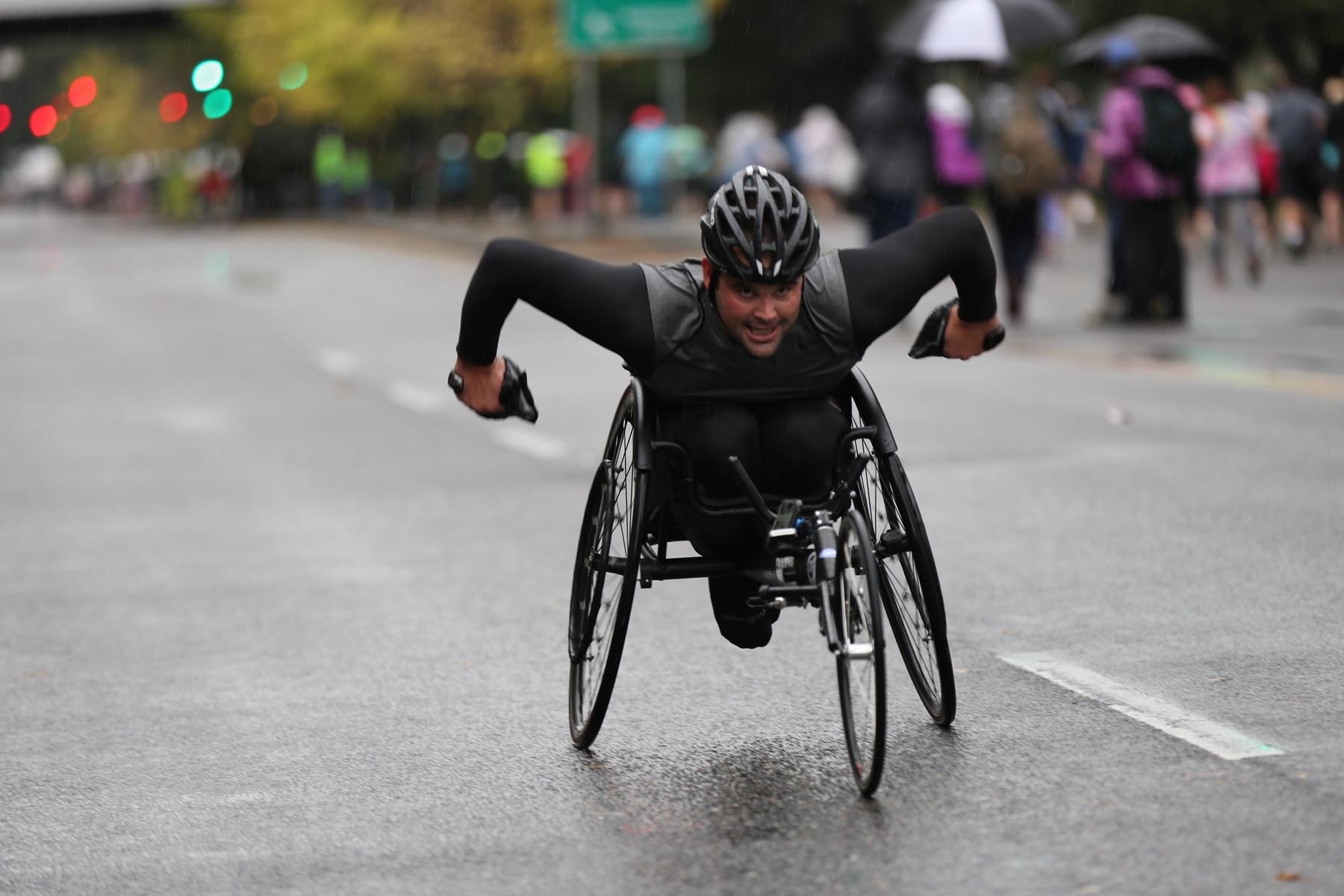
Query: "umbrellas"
1059 14 1221 69
878 0 1074 62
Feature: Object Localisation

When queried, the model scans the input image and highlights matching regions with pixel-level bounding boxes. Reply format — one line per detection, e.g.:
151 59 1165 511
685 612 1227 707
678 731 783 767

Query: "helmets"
699 164 821 284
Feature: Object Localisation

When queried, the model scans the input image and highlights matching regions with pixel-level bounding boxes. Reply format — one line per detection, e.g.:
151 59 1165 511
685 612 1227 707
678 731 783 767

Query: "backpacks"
1121 77 1201 174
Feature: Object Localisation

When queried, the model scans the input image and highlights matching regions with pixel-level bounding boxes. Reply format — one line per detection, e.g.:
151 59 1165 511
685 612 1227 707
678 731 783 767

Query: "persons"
447 164 1004 649
0 0 1344 327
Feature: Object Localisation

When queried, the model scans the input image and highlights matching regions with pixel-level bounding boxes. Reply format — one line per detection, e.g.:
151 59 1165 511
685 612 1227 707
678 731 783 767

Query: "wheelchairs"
568 354 960 799
